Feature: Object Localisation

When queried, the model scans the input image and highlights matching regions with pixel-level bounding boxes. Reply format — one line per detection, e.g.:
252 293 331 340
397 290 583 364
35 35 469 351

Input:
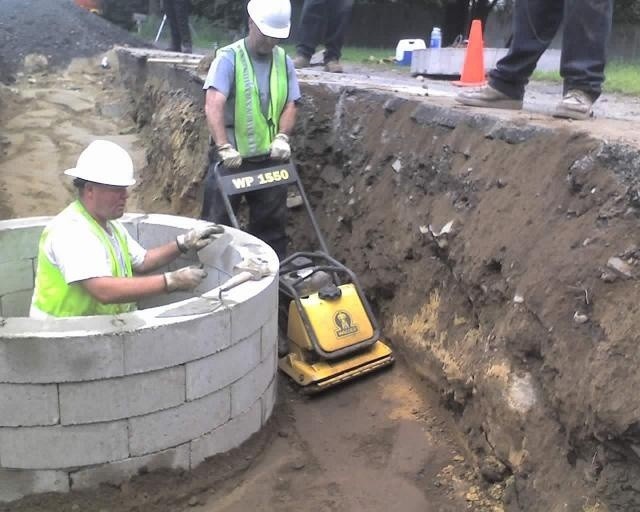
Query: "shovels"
197 232 234 269
154 271 252 318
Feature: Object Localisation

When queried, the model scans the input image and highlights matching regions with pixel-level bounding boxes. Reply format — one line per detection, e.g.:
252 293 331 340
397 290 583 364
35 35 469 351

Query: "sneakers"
324 61 343 73
555 89 593 120
455 85 523 110
292 56 309 69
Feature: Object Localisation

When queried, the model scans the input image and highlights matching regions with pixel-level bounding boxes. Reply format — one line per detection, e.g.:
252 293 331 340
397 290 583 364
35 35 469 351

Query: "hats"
247 0 292 39
64 139 136 186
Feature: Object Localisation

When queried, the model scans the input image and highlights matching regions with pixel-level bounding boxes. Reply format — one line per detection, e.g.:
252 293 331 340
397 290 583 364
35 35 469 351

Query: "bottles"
431 27 442 49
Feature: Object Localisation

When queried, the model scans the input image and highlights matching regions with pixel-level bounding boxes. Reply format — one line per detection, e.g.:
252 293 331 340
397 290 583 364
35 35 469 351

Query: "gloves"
164 262 208 293
176 221 224 254
266 133 291 161
215 143 242 169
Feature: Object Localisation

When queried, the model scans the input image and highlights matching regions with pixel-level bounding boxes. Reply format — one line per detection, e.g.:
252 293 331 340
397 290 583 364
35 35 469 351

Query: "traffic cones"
452 21 488 89
430 28 441 45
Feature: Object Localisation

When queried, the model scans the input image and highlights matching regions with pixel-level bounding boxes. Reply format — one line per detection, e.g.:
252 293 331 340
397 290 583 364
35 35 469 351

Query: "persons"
29 139 224 317
456 0 614 120
293 0 356 73
161 0 195 53
202 0 302 357
